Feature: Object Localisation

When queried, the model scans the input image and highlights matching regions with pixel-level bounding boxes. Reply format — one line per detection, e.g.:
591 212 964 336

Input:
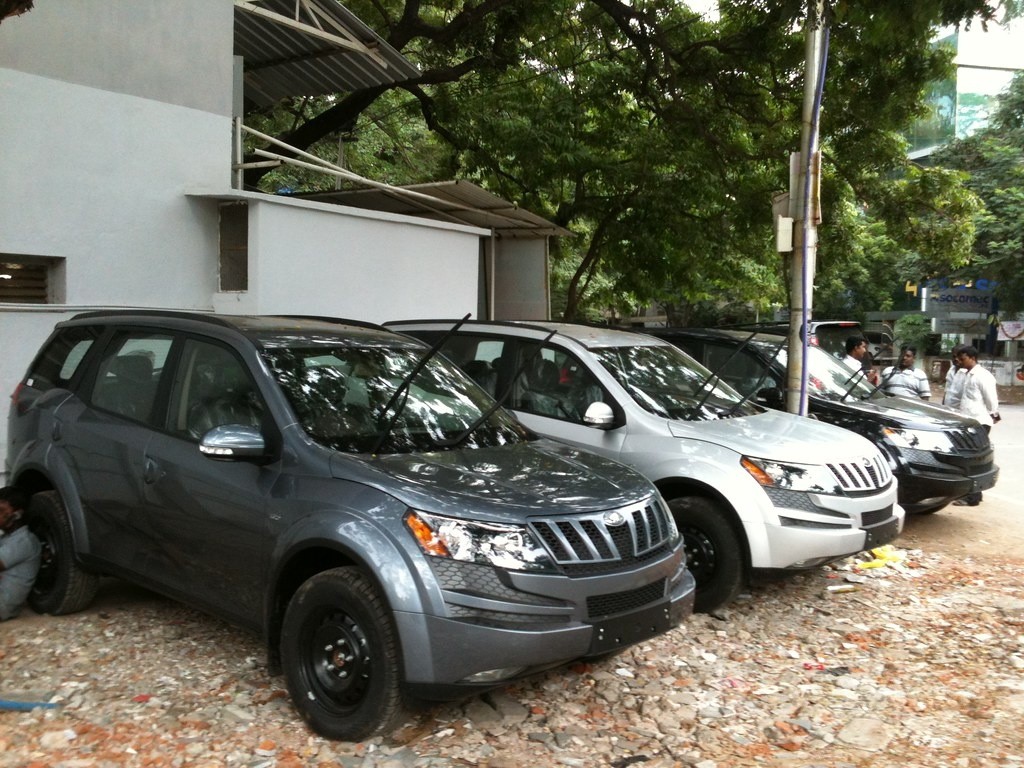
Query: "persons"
942 344 998 505
842 335 878 385
879 342 931 402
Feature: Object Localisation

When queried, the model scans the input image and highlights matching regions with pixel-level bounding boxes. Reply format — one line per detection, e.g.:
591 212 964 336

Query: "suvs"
7 309 699 745
560 319 1002 520
343 313 907 615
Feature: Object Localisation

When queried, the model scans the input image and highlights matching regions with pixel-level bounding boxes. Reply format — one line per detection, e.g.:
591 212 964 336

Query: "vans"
729 320 879 389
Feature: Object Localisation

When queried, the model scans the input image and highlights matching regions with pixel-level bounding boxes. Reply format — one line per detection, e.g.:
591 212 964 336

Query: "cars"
862 331 903 364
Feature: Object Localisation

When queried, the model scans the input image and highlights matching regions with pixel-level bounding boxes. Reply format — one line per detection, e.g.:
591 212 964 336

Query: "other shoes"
952 492 983 507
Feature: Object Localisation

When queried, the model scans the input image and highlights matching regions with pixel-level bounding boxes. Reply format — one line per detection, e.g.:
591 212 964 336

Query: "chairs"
709 352 737 391
187 360 265 439
566 365 602 415
498 359 580 409
295 365 376 437
736 359 769 401
102 355 159 420
